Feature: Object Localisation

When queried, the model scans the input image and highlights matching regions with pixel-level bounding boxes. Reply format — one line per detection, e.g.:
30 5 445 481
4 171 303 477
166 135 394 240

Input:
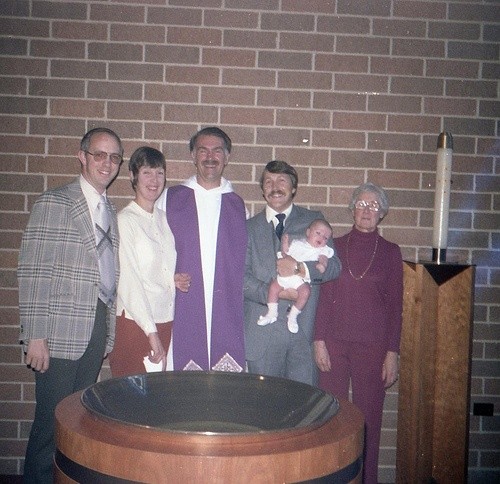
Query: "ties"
94 195 118 308
276 214 286 240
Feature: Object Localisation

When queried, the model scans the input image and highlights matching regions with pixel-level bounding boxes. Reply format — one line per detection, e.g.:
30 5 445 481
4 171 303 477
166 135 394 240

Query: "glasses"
84 150 123 165
353 200 381 212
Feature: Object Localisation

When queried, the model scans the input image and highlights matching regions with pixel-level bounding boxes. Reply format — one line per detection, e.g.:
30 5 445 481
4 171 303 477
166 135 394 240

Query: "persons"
256 219 336 334
308 182 403 484
18 128 123 484
153 126 250 373
244 160 343 384
107 146 192 378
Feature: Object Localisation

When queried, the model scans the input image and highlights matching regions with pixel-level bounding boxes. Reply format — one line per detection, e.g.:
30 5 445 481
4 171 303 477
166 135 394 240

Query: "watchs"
294 260 302 274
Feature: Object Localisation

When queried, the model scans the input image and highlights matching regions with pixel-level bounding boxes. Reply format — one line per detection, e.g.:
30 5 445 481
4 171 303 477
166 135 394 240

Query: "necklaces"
345 229 379 281
